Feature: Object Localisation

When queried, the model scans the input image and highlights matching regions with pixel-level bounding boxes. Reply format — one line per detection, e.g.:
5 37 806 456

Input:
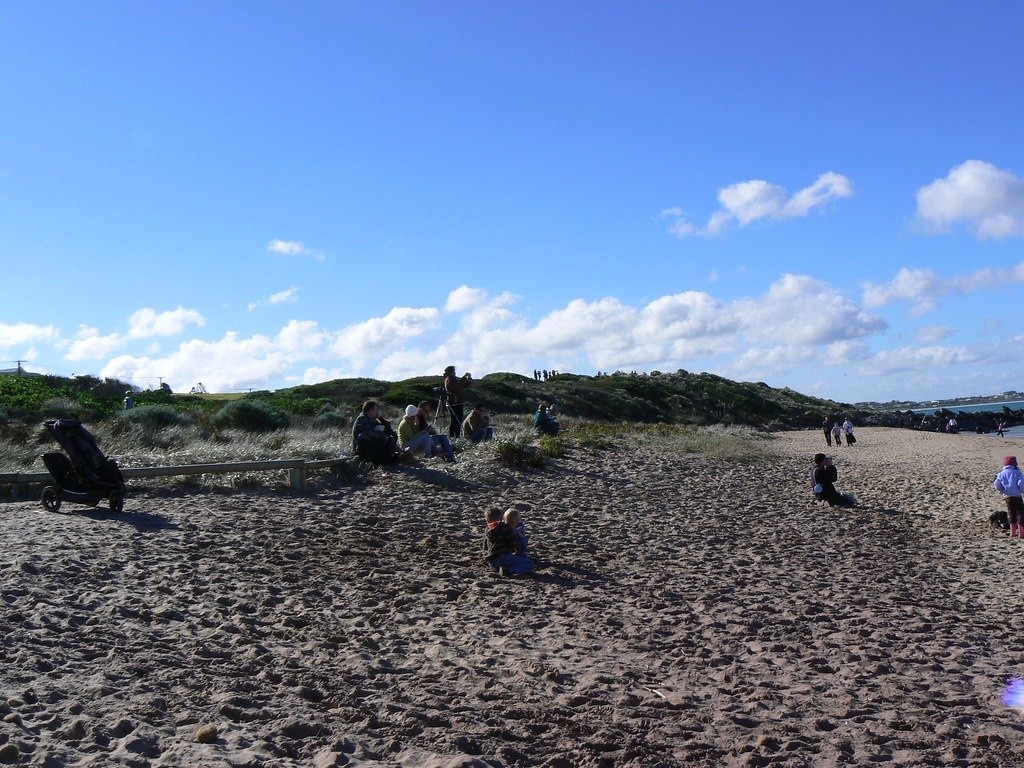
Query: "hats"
1004 456 1018 466
845 416 849 420
443 366 456 372
405 404 419 418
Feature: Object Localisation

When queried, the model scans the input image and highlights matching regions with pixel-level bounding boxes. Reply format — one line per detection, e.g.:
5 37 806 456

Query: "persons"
398 401 459 465
994 455 1024 539
463 402 493 443
631 370 638 375
995 421 1006 437
484 507 536 577
533 368 559 382
945 417 958 434
842 417 857 446
811 452 840 500
123 390 134 410
822 416 842 447
533 402 559 436
444 365 472 440
350 400 412 468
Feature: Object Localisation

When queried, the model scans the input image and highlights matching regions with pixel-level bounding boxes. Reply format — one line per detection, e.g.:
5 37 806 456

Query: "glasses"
476 409 485 411
422 408 430 412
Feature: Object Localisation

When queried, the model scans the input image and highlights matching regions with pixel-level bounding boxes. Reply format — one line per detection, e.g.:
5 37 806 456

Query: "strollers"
40 416 126 514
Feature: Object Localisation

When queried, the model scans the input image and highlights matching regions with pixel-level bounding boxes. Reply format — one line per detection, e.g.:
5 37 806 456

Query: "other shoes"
448 457 462 464
499 565 508 577
455 438 467 442
449 436 456 441
426 454 435 458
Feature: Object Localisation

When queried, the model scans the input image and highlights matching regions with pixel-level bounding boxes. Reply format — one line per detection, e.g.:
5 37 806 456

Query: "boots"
1010 523 1018 537
1017 522 1024 539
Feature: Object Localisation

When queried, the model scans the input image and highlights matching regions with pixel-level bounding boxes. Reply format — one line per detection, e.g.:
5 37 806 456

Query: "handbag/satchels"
814 483 823 494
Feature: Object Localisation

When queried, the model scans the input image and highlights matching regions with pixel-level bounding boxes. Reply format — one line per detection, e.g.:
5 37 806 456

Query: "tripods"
432 393 461 433
